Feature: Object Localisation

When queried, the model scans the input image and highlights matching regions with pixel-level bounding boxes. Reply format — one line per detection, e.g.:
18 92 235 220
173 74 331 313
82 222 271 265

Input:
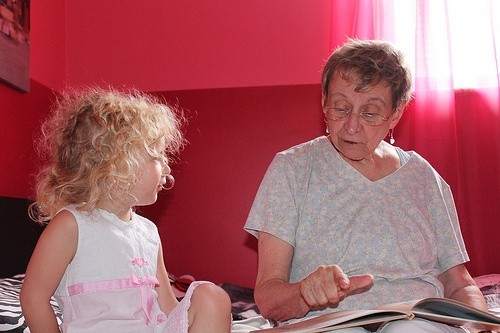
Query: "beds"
0 195 500 332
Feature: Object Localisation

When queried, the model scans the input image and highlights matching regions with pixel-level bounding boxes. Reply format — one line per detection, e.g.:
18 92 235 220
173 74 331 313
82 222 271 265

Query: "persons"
19 80 232 333
243 33 499 333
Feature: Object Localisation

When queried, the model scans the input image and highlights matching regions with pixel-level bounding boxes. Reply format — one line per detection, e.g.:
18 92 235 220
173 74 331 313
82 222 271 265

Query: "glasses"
323 91 400 126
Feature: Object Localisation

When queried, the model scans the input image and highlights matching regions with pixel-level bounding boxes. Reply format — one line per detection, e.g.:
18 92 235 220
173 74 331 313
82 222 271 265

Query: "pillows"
0 273 62 332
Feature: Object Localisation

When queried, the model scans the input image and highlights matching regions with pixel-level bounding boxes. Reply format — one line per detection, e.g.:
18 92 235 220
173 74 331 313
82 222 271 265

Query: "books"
248 297 500 333
